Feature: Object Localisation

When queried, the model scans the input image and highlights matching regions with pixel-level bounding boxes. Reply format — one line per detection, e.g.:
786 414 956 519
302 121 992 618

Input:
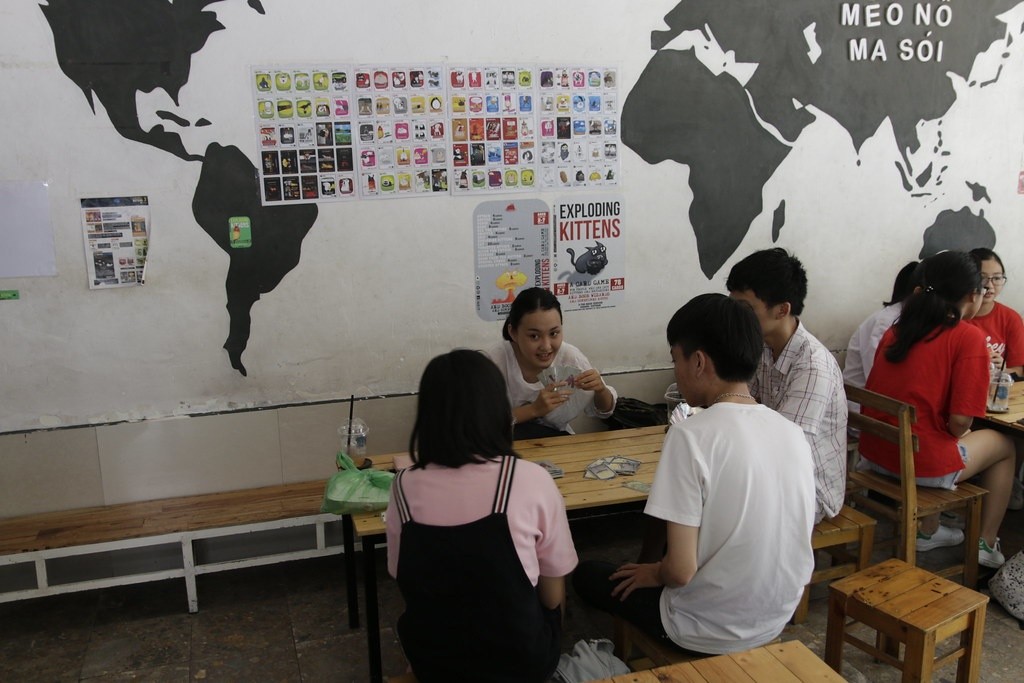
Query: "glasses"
982 277 1007 286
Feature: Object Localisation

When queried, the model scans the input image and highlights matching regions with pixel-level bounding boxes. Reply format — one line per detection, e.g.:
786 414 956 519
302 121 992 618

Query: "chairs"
842 382 991 588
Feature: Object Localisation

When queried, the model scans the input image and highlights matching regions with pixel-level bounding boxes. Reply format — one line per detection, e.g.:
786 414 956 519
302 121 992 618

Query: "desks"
342 422 669 683
981 367 1024 432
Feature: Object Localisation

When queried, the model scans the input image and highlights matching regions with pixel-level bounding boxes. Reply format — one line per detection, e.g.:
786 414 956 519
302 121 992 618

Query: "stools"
567 504 988 683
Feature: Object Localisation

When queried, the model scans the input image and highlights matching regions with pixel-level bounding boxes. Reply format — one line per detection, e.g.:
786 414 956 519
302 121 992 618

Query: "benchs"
1 475 386 613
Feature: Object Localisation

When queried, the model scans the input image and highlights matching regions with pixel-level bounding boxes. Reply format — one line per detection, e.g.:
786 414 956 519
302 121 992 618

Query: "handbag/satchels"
987 550 1024 623
319 451 397 514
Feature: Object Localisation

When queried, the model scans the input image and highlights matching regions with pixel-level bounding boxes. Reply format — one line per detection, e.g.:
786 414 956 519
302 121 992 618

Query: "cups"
337 426 369 468
986 377 1014 411
664 392 686 428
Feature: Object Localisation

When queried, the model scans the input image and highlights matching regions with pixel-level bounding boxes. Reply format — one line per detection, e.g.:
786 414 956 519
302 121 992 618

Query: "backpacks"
552 638 630 683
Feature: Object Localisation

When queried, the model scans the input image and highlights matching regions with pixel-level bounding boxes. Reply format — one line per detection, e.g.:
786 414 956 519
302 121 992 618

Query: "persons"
478 286 618 442
566 294 817 658
725 247 849 525
843 248 1024 567
388 347 579 683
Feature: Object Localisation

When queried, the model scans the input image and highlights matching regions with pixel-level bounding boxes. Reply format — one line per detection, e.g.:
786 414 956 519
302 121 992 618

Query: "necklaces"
712 393 755 404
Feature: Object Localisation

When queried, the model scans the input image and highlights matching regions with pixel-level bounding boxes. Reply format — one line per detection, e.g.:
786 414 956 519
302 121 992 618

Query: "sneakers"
976 535 1004 568
915 521 966 553
